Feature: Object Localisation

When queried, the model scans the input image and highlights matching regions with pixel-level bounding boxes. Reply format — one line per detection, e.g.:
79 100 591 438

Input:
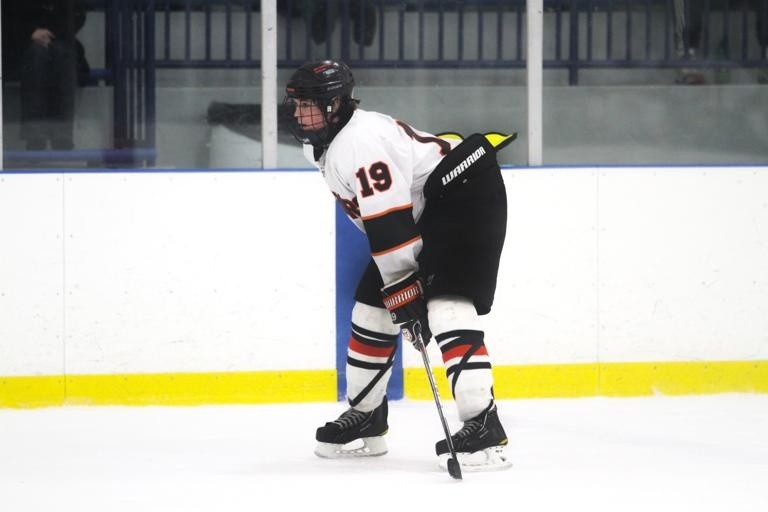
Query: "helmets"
285 60 354 99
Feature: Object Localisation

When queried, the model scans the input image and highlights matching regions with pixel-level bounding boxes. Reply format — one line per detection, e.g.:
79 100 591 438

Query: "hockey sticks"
417 326 462 480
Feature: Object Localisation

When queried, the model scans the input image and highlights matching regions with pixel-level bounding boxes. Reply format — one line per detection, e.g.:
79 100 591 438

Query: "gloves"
380 270 432 351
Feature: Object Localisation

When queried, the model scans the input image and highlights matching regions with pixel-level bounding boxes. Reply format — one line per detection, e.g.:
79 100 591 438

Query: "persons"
7 0 88 149
283 61 509 456
666 0 768 85
307 0 376 48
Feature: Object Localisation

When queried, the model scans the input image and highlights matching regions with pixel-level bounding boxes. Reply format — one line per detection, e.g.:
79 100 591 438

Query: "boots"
316 395 388 444
436 386 508 456
